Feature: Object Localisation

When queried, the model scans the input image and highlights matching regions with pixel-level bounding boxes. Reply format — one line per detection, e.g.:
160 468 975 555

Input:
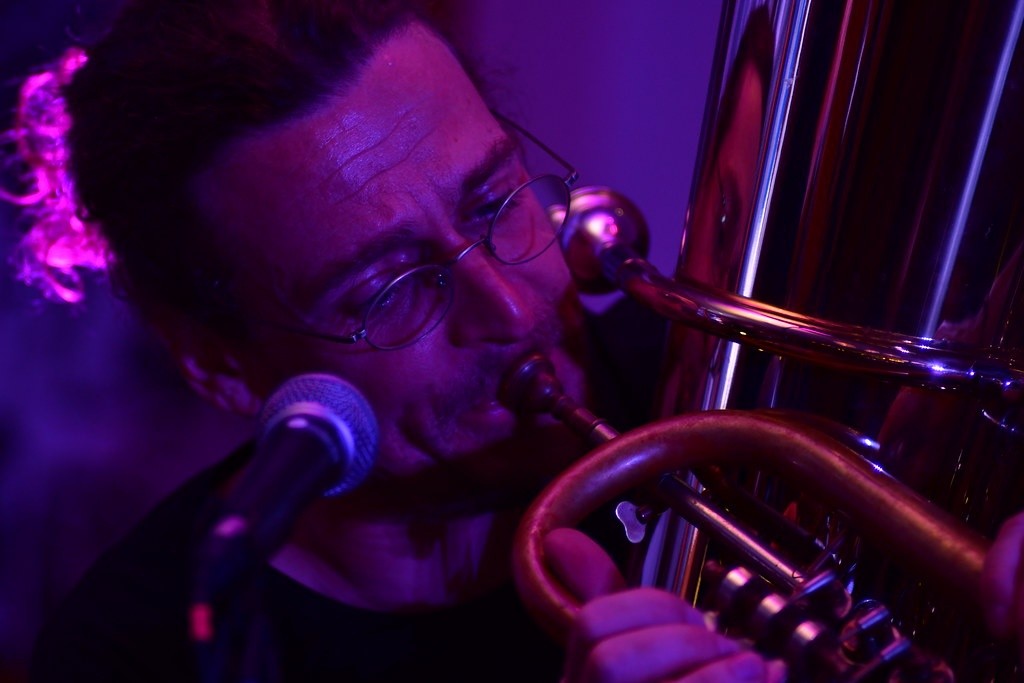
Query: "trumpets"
494 350 1024 682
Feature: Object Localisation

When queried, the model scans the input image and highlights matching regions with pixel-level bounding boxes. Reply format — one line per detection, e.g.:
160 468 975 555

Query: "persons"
29 1 871 683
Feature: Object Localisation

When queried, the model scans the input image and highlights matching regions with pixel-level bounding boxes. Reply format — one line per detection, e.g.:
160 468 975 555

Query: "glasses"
165 108 580 351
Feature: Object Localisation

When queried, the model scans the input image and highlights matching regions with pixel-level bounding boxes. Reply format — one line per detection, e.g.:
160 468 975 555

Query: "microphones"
204 374 379 566
